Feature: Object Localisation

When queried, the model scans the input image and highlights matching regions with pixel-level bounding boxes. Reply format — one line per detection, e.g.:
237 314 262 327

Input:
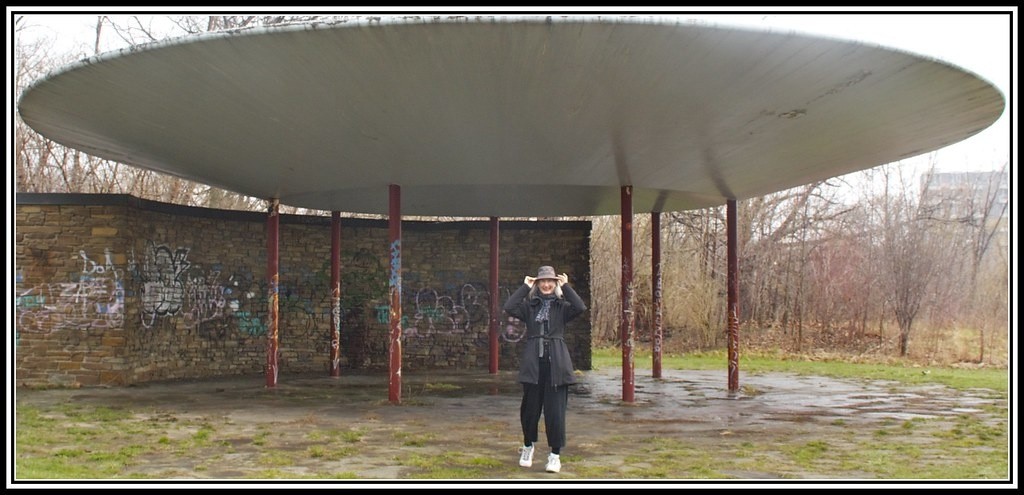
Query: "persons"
503 266 588 473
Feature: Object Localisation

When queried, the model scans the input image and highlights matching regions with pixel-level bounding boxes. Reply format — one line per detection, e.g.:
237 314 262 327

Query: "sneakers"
545 453 561 472
518 444 535 467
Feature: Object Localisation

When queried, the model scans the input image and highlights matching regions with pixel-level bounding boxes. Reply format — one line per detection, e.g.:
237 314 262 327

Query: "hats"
535 266 560 280
